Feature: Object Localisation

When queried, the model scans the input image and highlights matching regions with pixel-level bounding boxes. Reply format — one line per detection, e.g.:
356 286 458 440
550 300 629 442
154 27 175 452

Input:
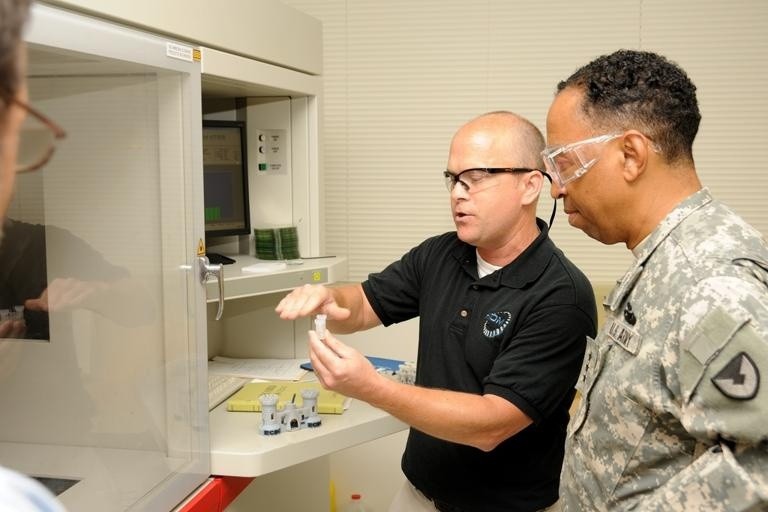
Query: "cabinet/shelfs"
0 0 225 512
200 43 417 478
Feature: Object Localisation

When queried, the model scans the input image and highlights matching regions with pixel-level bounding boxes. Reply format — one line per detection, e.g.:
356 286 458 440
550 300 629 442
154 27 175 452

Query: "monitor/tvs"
202 118 252 265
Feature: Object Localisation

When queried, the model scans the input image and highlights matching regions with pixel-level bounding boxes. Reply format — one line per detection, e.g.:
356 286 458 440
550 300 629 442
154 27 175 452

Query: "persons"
0 1 71 510
0 217 163 382
540 47 768 512
274 109 599 512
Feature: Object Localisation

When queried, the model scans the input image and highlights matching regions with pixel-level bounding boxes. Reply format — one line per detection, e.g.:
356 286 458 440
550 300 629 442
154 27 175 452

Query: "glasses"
443 166 533 194
5 94 65 174
539 131 662 188
